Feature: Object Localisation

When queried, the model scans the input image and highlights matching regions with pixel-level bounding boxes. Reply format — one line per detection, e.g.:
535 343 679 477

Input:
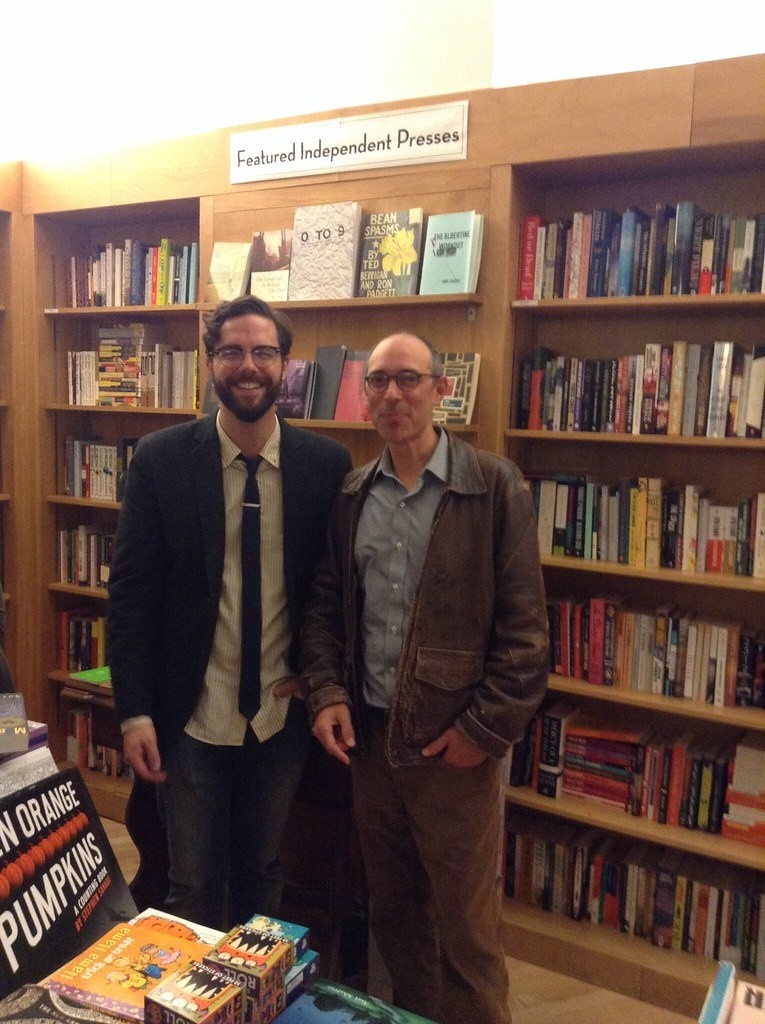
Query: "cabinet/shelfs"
0 53 765 1018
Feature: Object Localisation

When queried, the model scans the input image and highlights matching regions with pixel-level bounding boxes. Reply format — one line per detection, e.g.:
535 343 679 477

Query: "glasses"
364 370 440 393
209 345 281 369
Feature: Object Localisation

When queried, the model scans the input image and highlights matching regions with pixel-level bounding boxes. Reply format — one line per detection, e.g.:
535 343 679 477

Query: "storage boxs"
144 914 321 1024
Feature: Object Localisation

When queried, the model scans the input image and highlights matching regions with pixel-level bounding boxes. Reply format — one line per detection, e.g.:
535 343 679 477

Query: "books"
42 200 765 1024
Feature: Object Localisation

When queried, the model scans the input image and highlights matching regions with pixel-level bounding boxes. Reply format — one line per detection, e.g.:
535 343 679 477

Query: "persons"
292 332 553 1024
105 297 357 990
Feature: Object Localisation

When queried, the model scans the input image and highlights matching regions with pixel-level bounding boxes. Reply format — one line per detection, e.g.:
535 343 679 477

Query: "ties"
236 452 262 721
